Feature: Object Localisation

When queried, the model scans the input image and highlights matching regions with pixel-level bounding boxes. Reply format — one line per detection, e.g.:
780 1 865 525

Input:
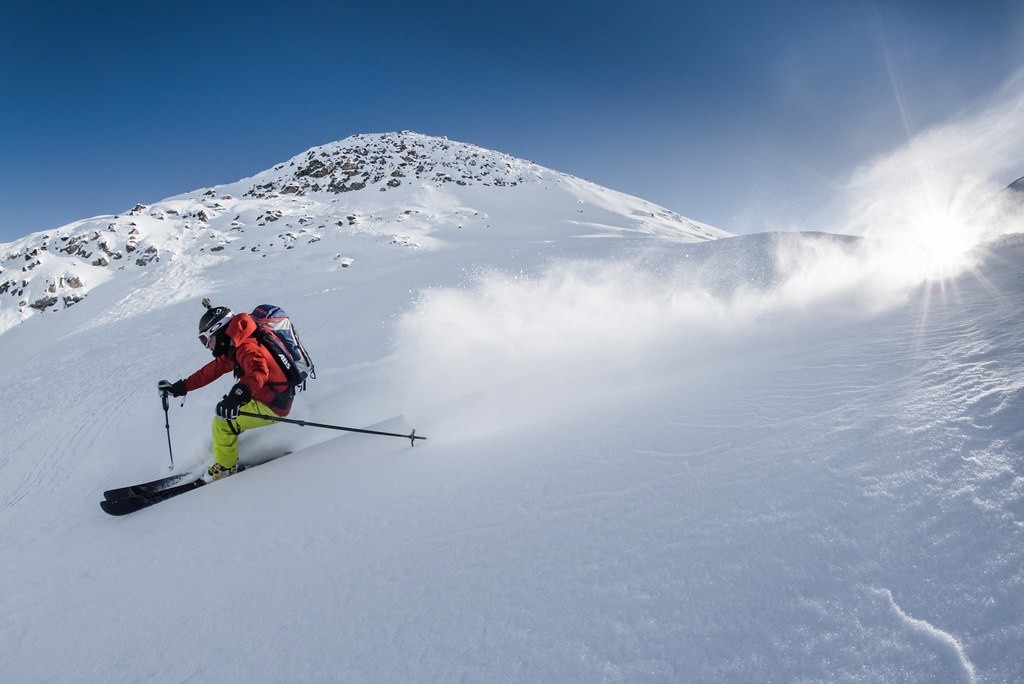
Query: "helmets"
199 307 234 348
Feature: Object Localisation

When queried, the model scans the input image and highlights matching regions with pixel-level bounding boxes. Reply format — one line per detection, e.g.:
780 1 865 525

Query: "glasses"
199 332 212 348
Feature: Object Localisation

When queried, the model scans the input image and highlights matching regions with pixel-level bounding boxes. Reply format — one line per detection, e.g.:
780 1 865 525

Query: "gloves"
158 379 187 397
216 383 251 420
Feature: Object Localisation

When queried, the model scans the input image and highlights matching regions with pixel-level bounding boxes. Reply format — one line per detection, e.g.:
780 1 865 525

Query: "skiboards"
98 445 293 519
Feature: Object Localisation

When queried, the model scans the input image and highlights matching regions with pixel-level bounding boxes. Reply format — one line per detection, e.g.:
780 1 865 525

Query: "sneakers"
203 457 239 482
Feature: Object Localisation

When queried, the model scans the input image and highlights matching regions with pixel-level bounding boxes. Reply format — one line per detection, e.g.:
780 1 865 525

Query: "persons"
157 297 316 486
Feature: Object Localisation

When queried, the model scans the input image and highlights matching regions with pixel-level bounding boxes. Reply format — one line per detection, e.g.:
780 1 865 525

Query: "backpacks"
234 304 316 399
